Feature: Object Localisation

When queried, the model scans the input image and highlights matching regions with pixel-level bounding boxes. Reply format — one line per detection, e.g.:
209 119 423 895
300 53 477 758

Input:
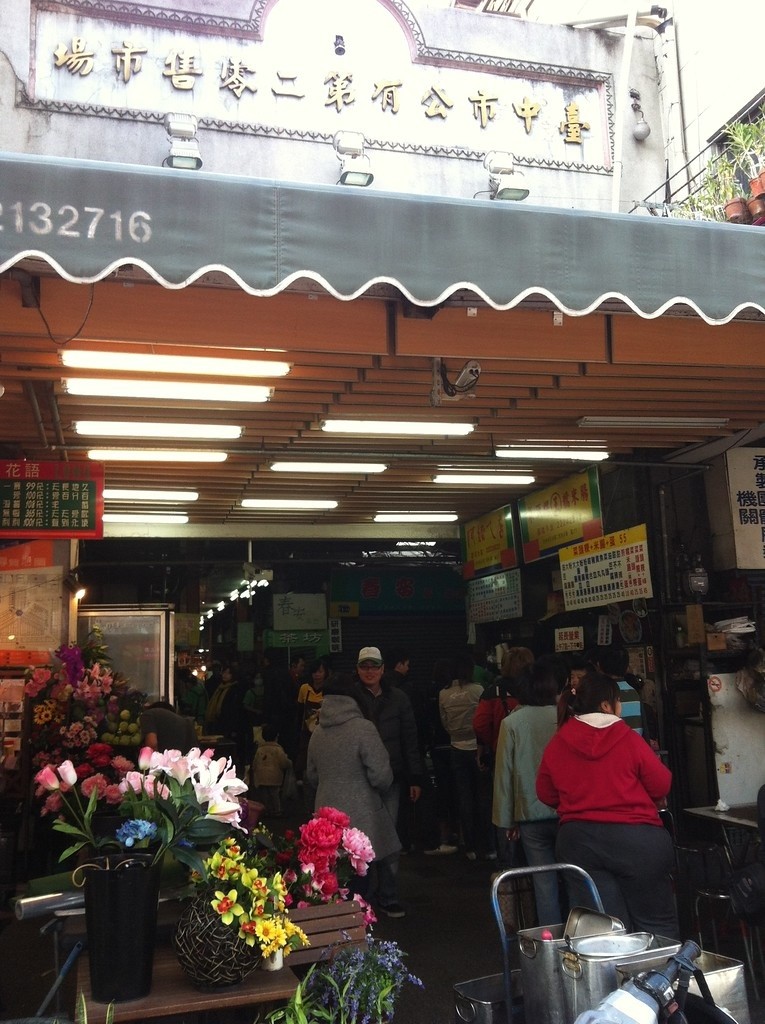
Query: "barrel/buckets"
517 905 750 1024
452 968 525 1024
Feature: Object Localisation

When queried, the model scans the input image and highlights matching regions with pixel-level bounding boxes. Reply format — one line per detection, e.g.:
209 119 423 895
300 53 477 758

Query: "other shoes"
399 843 416 855
423 844 458 855
484 848 498 860
380 903 406 917
264 811 270 818
274 811 290 818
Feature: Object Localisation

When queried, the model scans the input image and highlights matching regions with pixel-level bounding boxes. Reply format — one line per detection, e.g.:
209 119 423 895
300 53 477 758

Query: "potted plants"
681 103 765 225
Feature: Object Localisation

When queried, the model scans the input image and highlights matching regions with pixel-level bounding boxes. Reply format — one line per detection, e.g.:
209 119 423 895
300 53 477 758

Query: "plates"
632 599 648 618
619 611 642 644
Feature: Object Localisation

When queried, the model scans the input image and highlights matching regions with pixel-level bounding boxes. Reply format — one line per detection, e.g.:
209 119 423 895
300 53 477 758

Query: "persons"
179 646 644 932
142 701 189 754
537 670 679 940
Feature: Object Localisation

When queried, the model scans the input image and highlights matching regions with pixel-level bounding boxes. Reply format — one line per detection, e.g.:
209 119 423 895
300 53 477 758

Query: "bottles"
675 626 685 647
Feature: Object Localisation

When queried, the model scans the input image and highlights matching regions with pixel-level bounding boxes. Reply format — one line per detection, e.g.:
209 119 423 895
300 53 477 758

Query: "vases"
84 851 159 1003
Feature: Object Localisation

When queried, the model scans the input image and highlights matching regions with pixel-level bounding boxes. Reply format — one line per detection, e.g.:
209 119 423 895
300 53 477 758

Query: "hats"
358 647 382 665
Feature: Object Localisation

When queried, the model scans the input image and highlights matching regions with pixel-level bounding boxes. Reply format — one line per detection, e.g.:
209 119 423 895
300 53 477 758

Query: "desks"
74 941 303 1024
684 801 765 969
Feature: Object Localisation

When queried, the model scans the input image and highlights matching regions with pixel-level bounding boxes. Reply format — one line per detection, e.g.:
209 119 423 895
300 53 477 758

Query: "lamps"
162 112 202 170
483 151 529 200
63 565 86 601
632 104 650 140
61 348 728 526
333 130 374 185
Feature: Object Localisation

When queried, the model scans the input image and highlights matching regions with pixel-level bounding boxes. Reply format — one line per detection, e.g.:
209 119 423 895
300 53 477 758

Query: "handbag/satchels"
280 769 300 804
490 838 536 935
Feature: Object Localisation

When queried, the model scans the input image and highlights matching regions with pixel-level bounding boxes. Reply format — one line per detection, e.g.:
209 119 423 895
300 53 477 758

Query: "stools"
695 884 765 1001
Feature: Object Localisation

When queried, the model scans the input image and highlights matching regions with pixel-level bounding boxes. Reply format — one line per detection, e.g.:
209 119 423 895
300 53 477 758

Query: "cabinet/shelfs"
658 600 759 845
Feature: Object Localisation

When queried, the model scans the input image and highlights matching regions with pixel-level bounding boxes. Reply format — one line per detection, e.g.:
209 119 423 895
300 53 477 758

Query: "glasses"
359 664 383 670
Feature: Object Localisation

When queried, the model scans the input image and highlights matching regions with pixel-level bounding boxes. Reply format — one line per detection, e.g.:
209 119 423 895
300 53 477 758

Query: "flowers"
21 622 428 1024
